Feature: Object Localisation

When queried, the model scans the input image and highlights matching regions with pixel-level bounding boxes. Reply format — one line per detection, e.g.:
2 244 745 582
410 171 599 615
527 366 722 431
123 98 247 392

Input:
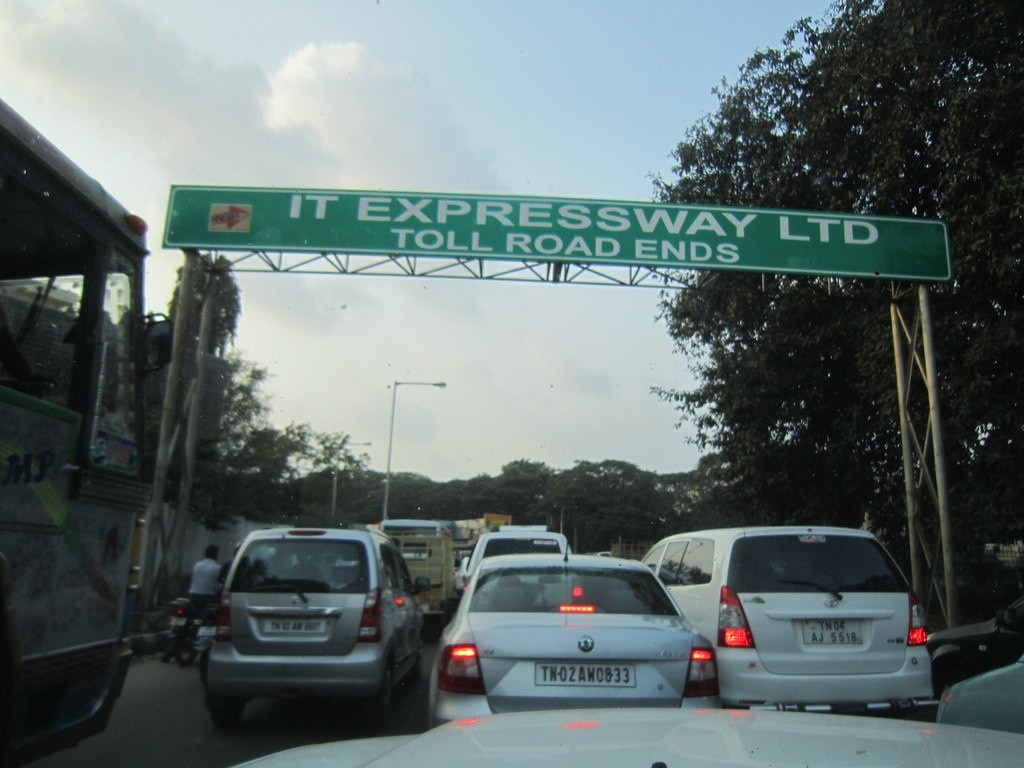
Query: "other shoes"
161 649 174 663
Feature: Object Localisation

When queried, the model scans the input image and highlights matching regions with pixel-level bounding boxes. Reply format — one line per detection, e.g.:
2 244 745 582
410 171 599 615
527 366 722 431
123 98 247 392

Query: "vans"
640 525 941 713
199 521 431 721
469 521 574 587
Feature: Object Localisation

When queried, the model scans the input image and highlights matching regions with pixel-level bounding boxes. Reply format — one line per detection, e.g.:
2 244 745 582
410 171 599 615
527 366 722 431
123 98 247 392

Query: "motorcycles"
164 591 212 669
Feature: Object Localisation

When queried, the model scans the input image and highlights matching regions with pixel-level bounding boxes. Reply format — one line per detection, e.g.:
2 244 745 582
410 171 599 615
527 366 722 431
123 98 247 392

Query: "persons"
162 545 227 663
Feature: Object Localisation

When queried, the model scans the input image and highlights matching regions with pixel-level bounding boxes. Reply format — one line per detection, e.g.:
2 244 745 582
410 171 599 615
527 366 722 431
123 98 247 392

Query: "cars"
433 555 722 723
930 646 1024 735
919 592 1024 684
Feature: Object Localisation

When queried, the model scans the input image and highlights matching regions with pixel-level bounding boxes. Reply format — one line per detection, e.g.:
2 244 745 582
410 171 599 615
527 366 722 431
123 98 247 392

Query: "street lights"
382 380 447 521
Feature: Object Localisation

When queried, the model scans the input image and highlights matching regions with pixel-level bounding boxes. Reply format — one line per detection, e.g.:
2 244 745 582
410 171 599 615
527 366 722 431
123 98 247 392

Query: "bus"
0 95 155 768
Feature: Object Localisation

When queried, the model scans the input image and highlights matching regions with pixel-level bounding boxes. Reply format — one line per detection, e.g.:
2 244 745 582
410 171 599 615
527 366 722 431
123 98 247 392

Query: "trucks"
366 514 515 624
